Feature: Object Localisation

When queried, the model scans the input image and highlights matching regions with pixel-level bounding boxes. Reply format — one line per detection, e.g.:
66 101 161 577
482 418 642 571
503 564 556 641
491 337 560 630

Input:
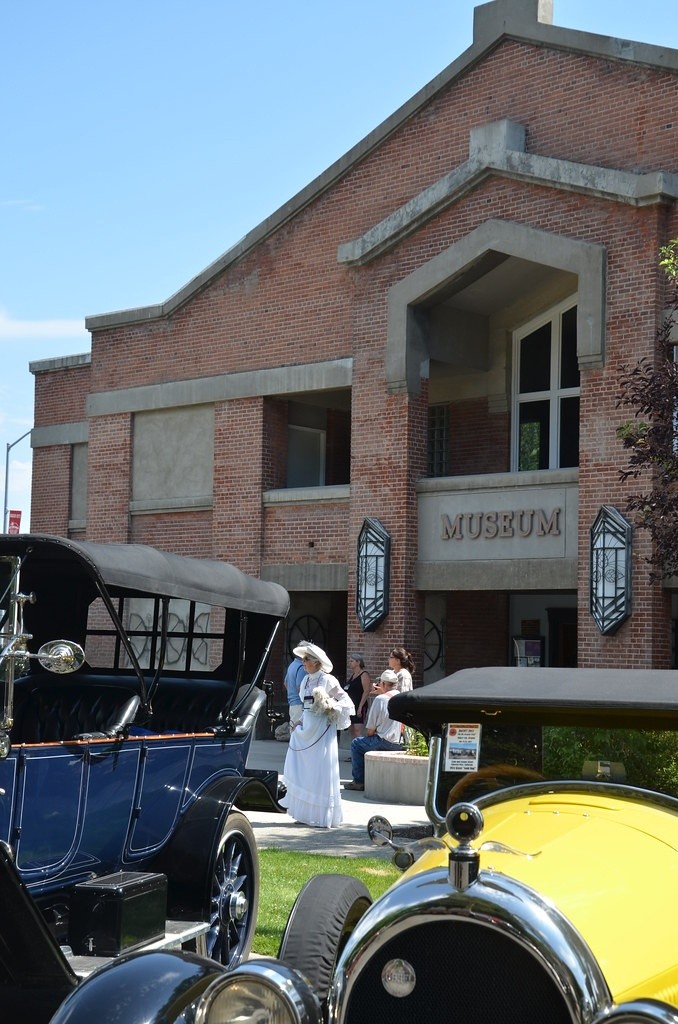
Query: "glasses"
389 655 395 659
304 656 310 662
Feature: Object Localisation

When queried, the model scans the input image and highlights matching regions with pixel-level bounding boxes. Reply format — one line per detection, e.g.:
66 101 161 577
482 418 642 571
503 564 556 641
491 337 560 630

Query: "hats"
380 669 398 683
293 644 333 674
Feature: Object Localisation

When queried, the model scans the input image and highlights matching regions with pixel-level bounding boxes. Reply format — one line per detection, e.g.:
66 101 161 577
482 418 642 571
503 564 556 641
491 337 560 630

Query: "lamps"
355 517 391 633
588 506 633 637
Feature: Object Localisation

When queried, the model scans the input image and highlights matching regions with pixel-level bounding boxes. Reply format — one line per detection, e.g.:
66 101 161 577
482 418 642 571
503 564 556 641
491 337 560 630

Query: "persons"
277 646 356 829
344 653 371 762
283 640 315 737
344 669 403 791
369 647 414 750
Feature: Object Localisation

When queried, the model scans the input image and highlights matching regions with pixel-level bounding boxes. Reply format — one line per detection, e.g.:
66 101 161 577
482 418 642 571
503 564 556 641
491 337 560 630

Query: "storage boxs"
71 871 168 958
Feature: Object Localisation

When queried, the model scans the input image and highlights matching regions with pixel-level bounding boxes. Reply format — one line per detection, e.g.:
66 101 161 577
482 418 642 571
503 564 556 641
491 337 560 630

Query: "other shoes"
343 756 351 762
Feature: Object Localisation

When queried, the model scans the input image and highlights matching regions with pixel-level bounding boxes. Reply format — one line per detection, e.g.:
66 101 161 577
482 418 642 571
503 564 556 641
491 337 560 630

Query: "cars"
3 530 293 971
49 667 678 1024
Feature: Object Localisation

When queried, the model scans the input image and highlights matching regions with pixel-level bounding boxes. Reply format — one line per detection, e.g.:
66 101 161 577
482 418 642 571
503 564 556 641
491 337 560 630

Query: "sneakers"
344 781 364 791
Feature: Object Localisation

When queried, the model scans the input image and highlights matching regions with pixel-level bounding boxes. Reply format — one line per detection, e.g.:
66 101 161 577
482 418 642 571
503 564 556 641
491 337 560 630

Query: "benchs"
1 671 268 743
